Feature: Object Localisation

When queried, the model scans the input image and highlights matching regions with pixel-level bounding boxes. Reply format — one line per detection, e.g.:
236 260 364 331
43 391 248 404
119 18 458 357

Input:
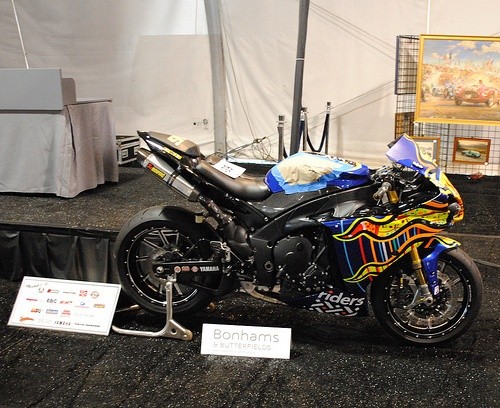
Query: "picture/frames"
452 136 491 164
409 136 441 165
414 33 500 126
395 36 419 95
394 112 414 139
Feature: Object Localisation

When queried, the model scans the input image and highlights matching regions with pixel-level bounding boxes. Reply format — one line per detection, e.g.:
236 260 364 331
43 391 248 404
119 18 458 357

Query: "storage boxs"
116 135 140 165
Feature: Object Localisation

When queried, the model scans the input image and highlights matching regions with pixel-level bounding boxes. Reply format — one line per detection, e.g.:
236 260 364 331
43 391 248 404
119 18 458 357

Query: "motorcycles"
112 130 484 351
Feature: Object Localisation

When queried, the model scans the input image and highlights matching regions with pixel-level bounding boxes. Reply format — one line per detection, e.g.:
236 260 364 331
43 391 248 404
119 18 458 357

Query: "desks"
0 68 119 198
